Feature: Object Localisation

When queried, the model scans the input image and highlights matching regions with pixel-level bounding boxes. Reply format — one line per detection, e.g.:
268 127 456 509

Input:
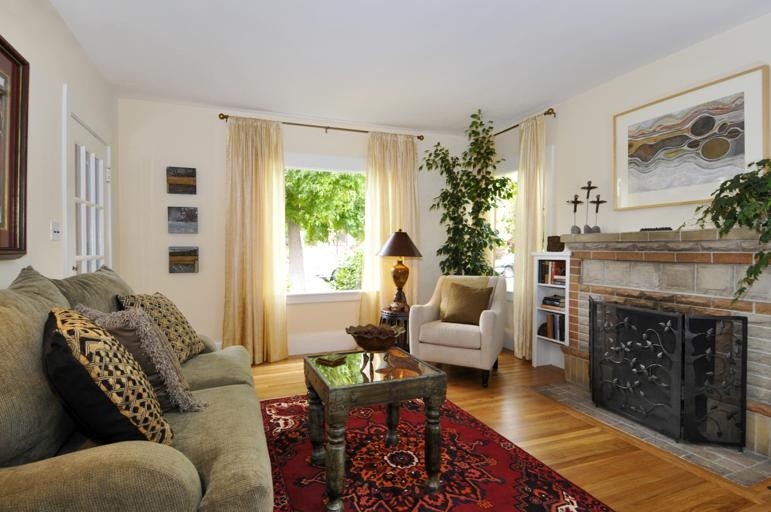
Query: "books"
539 260 565 341
316 354 348 367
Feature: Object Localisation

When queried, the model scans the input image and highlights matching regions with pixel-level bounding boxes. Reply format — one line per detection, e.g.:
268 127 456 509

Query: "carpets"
528 380 771 487
261 393 613 511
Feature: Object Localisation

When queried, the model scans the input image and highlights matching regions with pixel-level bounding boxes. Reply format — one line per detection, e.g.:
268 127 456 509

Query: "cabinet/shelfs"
529 251 570 369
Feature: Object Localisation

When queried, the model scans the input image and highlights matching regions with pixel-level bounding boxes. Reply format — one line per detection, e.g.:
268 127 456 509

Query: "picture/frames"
611 65 769 212
0 33 29 261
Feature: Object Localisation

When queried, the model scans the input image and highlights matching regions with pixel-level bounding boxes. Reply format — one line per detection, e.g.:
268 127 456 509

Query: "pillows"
0 266 72 468
441 283 493 325
116 291 205 364
49 265 135 314
73 301 210 414
42 306 175 445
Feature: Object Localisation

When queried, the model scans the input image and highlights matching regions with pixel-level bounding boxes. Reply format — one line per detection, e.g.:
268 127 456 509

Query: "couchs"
0 334 276 511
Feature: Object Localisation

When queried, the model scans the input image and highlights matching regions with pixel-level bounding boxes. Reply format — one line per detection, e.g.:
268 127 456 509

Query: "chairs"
409 273 506 389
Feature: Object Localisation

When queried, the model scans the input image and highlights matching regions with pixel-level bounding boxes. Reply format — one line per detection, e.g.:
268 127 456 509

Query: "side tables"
379 307 410 353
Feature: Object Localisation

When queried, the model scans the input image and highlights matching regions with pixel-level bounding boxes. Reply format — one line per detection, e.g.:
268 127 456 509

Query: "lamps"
377 228 423 312
